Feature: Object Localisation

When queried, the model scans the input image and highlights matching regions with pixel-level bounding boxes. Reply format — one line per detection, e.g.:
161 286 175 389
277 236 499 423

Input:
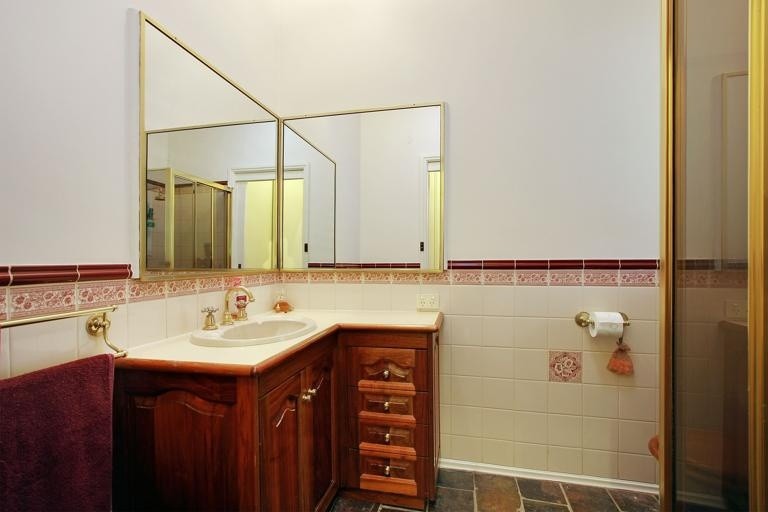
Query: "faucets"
225 286 256 313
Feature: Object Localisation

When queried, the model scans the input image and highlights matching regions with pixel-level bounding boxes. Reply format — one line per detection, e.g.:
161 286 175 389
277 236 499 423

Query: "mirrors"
280 102 445 273
138 11 280 281
146 118 278 272
283 124 336 270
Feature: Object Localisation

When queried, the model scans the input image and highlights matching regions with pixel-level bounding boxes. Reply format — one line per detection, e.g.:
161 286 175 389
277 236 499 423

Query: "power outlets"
725 299 746 319
416 293 440 309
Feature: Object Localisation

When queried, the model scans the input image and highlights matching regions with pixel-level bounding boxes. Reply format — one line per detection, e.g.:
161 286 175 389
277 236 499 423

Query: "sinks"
190 315 318 348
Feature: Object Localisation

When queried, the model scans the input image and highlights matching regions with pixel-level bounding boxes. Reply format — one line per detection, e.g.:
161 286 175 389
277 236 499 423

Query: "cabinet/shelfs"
110 332 339 512
717 323 748 507
339 332 442 512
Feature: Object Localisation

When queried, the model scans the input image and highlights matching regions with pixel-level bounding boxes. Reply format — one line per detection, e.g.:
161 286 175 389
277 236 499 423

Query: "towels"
0 353 115 512
